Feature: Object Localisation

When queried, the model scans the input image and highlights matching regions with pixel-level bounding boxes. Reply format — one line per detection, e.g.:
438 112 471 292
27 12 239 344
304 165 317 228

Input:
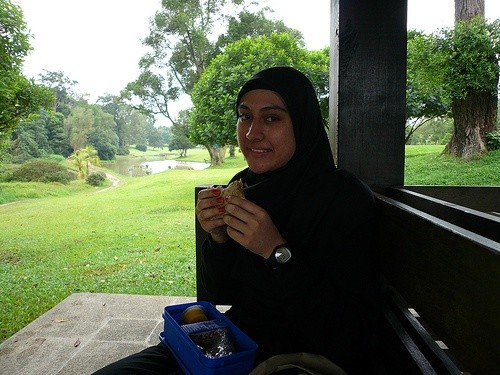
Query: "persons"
89 69 399 375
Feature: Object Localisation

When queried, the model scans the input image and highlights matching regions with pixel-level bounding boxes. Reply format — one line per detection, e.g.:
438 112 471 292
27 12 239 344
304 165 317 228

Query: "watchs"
266 242 292 270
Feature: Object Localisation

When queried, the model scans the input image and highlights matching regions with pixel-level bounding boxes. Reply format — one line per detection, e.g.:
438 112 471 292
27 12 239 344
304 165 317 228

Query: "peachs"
180 305 209 324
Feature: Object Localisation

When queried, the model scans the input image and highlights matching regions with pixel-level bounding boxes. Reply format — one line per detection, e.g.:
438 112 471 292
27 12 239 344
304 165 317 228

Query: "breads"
207 178 246 222
